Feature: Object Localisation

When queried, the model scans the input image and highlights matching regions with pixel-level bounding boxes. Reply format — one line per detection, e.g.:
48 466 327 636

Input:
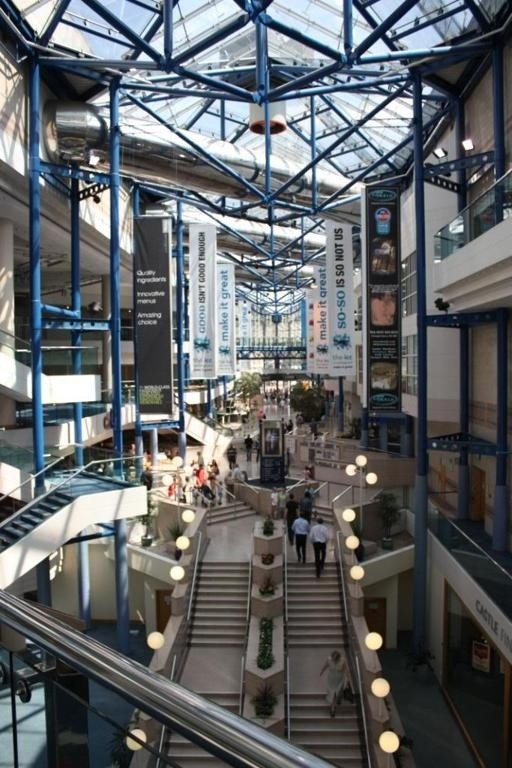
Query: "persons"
319 651 352 718
128 387 330 579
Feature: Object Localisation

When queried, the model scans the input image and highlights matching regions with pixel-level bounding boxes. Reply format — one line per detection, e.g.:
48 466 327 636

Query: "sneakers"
330 706 337 717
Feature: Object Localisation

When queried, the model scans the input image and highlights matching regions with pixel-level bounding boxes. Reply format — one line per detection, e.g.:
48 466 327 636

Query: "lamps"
462 139 477 157
432 148 448 164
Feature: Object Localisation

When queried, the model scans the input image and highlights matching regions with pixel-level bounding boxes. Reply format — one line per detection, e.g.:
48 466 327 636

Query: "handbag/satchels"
344 681 353 703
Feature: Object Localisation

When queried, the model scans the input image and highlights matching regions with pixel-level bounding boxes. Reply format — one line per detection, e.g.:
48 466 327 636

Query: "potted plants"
137 494 159 547
351 514 364 562
375 491 401 551
250 683 278 719
168 523 182 560
